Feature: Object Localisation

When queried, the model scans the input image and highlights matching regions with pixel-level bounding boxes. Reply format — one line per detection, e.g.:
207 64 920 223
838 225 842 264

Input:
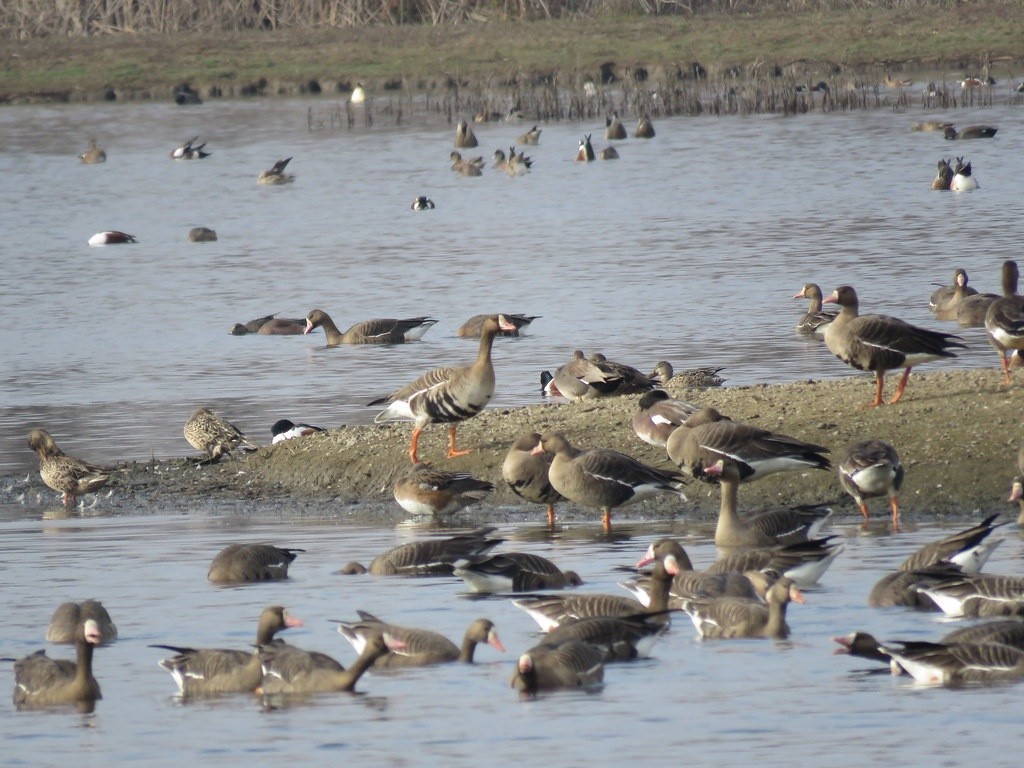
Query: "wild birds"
390 458 494 520
145 604 304 695
834 477 1024 692
701 458 833 548
209 542 305 588
230 309 540 348
330 611 505 675
451 554 583 597
931 156 977 194
256 626 404 698
796 73 1023 97
79 83 656 210
631 389 904 535
89 226 216 247
511 535 847 696
501 431 687 532
14 600 116 709
25 428 110 503
182 405 245 471
539 348 726 402
366 312 518 464
793 259 1024 406
910 119 998 140
332 525 505 579
